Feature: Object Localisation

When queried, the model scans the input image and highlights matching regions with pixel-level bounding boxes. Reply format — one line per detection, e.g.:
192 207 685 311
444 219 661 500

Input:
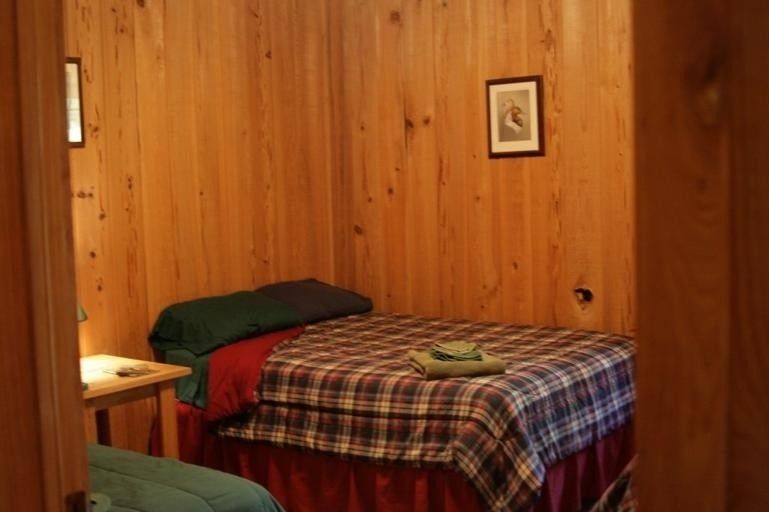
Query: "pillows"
254 278 374 322
153 291 301 359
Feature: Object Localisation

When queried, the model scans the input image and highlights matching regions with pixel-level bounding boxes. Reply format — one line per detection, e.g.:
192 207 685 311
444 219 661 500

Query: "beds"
150 310 635 511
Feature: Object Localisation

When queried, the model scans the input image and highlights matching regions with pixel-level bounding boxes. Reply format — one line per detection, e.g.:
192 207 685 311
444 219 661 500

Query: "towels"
405 340 506 380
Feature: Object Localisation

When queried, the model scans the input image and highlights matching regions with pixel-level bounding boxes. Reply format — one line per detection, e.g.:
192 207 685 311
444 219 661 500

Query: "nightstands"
80 353 194 461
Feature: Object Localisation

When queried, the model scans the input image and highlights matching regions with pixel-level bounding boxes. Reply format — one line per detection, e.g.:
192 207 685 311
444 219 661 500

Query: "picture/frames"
485 74 545 158
65 56 85 149
